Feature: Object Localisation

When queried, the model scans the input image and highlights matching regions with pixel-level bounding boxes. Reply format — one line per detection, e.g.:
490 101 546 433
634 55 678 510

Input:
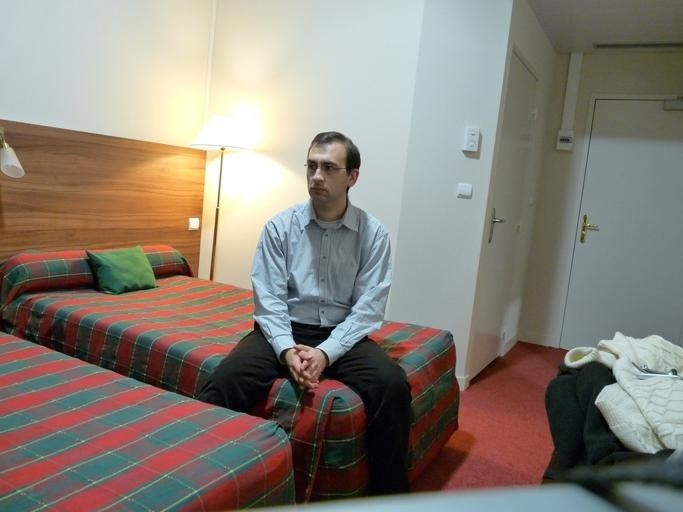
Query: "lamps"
0 127 25 177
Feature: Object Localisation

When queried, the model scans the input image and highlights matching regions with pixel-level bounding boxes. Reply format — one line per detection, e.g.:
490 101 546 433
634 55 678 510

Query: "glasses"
303 163 346 174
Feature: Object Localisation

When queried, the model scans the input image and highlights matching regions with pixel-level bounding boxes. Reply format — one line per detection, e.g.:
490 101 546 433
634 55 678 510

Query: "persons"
193 131 413 497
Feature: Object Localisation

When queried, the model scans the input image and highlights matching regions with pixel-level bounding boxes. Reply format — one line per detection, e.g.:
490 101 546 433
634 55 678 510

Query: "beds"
0 244 458 511
